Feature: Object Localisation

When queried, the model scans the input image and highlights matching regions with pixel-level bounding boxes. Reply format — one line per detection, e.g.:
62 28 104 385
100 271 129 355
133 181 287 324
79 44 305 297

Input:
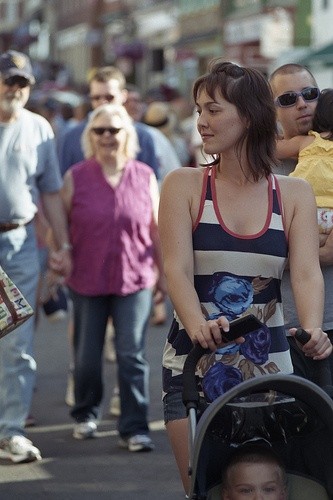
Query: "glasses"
4 77 31 90
91 92 115 103
90 126 123 136
274 86 321 109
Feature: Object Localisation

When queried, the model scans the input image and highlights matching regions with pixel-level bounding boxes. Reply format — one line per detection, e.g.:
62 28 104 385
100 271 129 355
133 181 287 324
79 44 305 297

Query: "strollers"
181 313 333 500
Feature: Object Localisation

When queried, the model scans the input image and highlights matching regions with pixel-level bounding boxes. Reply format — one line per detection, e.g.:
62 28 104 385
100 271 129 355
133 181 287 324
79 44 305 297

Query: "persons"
265 63 333 341
0 48 333 500
155 57 333 500
273 89 333 235
34 101 162 453
0 49 73 463
222 446 288 500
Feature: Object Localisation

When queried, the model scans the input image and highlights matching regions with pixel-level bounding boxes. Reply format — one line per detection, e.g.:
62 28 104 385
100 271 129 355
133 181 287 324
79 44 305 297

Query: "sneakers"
0 430 42 465
70 418 99 439
117 431 156 454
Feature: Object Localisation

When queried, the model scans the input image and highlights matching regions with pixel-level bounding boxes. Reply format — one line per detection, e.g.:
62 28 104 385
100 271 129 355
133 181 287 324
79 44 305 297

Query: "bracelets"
58 243 72 252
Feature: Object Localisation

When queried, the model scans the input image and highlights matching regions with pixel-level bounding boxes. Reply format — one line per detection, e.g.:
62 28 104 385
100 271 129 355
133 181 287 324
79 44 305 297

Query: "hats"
139 101 178 134
0 49 36 86
